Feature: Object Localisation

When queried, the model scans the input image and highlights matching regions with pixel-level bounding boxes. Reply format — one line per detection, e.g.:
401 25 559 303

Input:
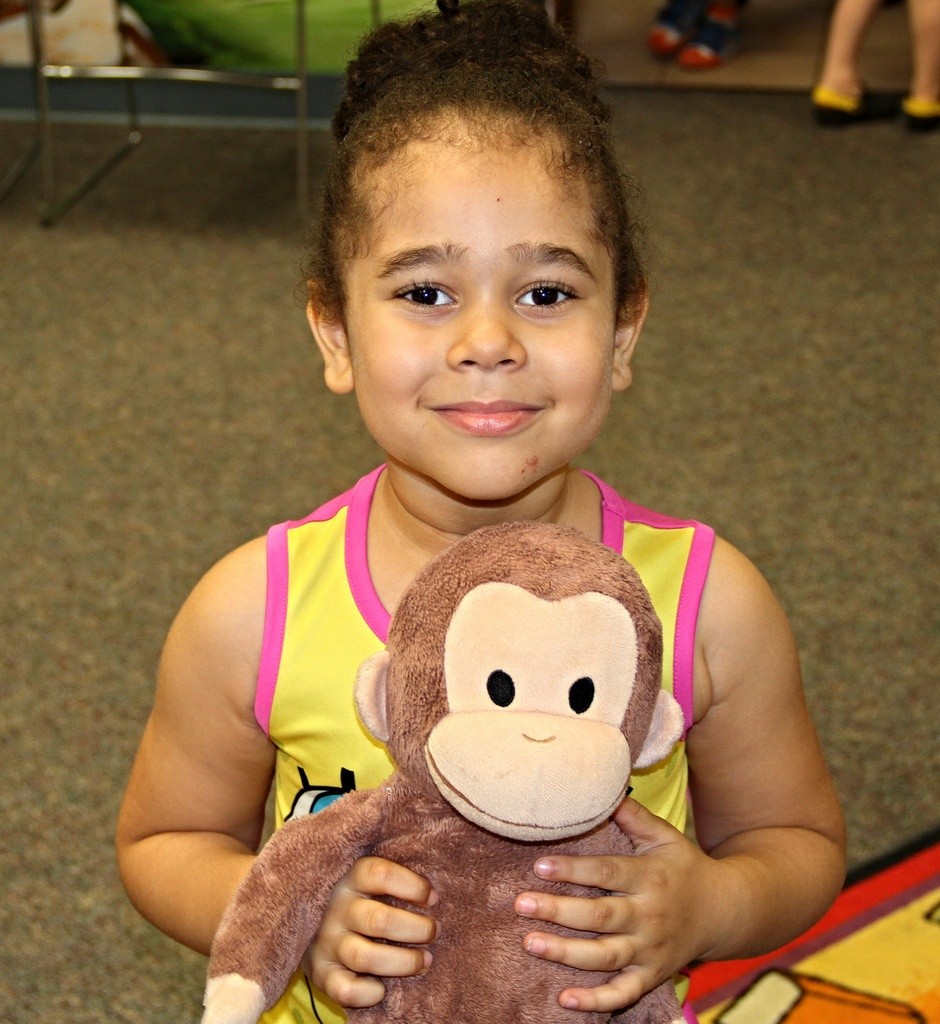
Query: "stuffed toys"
201 516 690 1024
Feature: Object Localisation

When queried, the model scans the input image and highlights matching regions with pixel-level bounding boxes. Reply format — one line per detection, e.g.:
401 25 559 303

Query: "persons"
642 1 748 70
115 20 848 1024
813 0 940 132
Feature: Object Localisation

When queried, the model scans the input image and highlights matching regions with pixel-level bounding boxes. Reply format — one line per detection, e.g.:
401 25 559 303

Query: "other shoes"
811 85 902 124
678 43 722 67
901 95 940 129
649 22 685 54
